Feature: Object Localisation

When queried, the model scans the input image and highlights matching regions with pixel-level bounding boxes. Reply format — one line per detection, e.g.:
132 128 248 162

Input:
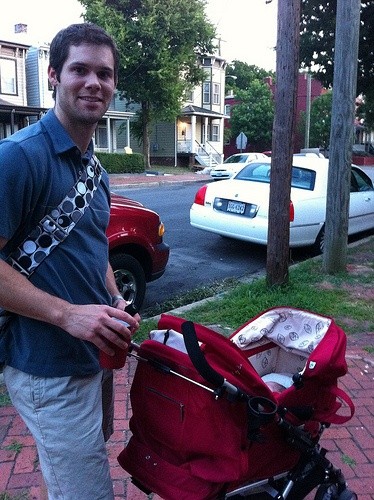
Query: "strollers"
116 306 355 499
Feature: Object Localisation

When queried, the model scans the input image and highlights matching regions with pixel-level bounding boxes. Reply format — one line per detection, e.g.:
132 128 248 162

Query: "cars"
190 154 374 255
209 152 269 182
107 191 171 315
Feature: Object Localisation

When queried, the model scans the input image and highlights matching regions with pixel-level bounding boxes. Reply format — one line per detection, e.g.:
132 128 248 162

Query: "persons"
0 23 142 500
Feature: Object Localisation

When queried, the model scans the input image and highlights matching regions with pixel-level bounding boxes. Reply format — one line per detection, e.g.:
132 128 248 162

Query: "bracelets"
111 295 124 305
